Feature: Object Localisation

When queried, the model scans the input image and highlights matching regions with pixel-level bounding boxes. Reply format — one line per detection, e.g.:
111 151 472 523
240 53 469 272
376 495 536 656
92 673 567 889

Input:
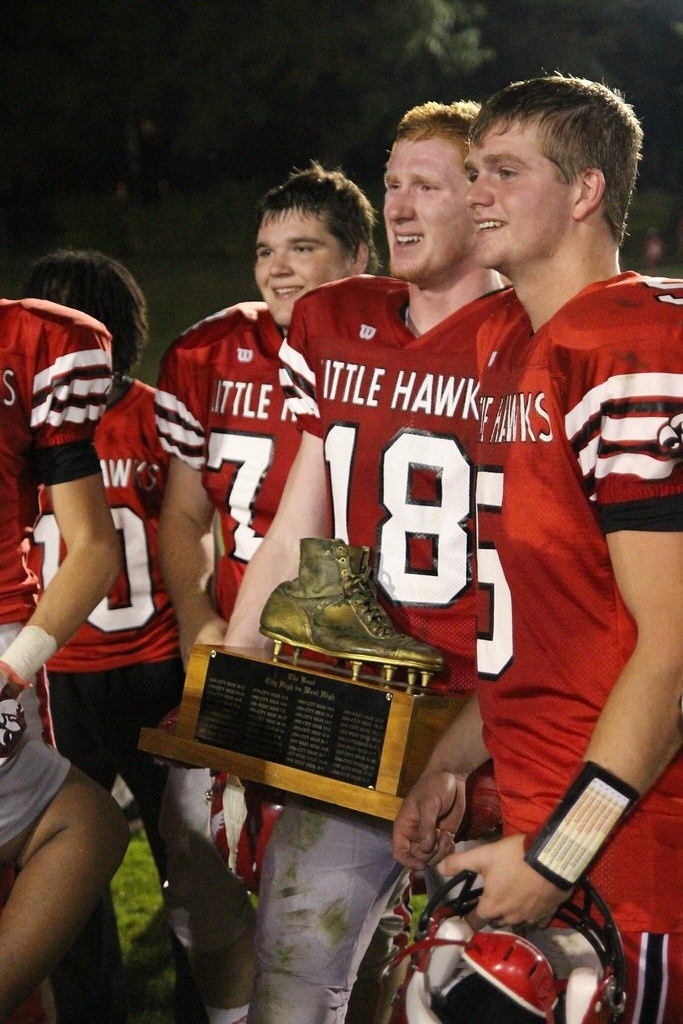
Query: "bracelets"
0 626 57 686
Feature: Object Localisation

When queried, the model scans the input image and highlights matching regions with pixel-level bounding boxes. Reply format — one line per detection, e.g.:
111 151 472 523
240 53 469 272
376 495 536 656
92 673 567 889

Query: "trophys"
139 538 472 820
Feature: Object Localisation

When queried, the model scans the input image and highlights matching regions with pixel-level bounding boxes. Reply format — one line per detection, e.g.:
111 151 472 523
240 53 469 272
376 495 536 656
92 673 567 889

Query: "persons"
156 166 378 896
0 296 130 1024
162 100 522 1024
394 78 683 1024
26 250 260 1024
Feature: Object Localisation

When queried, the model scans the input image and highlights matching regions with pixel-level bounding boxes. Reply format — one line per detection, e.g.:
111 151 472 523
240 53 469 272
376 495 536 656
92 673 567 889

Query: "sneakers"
258 537 445 686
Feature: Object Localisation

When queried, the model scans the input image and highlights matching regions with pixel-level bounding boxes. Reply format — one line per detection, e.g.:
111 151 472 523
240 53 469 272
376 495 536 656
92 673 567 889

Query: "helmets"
386 869 626 1024
210 775 285 899
